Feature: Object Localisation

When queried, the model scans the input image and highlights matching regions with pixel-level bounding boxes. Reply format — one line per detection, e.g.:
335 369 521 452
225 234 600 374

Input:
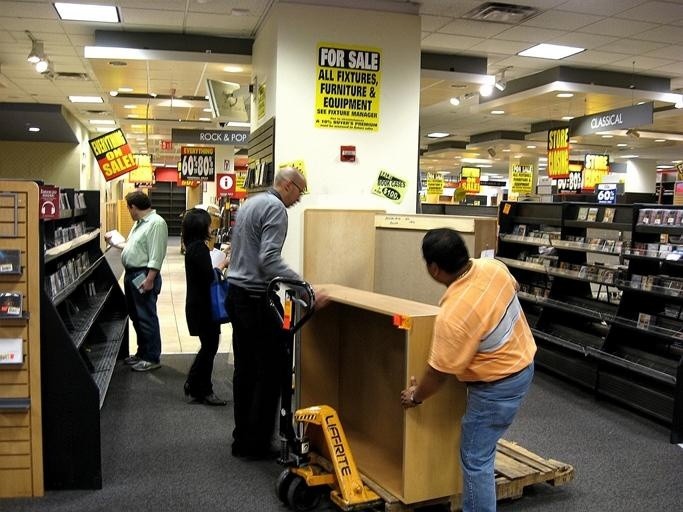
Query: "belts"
467 366 528 385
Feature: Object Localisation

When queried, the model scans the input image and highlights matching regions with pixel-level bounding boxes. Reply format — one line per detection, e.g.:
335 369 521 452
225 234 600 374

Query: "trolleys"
263 276 385 511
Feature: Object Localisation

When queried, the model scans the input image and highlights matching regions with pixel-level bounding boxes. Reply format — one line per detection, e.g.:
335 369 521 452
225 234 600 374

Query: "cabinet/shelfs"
495 199 683 444
296 208 498 505
38 182 130 490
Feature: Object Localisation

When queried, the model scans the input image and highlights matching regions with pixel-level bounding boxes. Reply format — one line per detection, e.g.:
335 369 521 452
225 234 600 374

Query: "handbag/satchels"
210 268 231 323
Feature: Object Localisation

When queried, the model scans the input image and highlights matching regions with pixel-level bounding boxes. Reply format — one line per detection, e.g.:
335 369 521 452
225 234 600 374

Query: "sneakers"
125 355 161 371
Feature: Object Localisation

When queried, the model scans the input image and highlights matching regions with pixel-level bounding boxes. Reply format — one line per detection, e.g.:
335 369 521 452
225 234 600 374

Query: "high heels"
184 382 227 405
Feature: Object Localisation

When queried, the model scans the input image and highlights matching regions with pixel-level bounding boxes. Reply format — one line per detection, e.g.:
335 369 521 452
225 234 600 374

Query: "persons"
182 207 231 406
223 167 329 461
120 191 169 371
401 228 537 512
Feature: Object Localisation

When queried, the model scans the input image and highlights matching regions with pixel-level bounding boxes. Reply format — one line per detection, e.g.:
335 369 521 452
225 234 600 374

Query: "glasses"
292 181 308 194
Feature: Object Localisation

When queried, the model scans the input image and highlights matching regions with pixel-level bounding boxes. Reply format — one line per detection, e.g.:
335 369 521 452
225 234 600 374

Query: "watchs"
410 391 423 405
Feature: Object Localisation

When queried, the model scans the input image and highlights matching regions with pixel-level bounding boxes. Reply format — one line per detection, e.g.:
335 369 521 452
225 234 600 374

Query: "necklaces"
455 264 470 279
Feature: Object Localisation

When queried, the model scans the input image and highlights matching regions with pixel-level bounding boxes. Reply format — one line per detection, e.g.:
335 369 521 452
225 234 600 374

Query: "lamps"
447 68 508 113
28 38 54 77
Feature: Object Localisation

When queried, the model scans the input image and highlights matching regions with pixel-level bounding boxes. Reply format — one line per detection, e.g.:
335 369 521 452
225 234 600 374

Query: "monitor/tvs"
206 78 248 122
